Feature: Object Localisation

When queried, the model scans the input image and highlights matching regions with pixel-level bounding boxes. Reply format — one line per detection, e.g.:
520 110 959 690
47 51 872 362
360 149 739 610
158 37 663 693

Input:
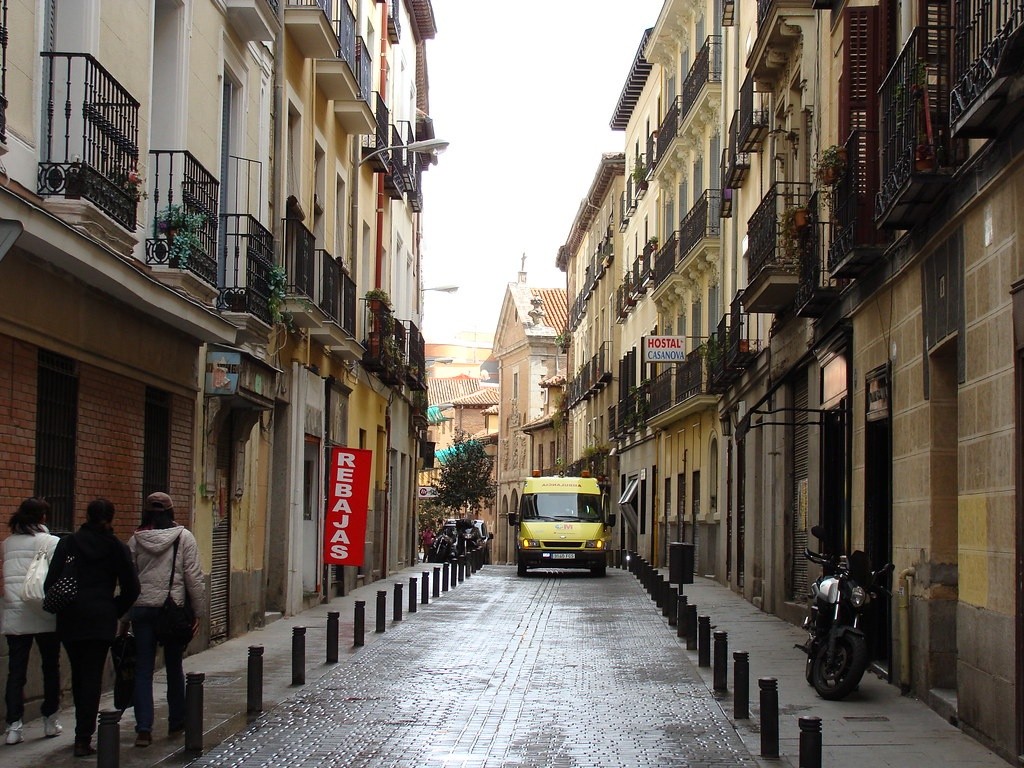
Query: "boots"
75 736 97 756
6 717 24 744
41 709 64 736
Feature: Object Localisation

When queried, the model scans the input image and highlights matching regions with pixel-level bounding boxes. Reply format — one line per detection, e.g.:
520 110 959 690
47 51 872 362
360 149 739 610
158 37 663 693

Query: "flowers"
917 133 927 160
115 170 149 201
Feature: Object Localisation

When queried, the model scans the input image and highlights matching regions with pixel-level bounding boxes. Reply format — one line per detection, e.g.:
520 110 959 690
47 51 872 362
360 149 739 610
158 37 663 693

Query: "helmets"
820 578 839 603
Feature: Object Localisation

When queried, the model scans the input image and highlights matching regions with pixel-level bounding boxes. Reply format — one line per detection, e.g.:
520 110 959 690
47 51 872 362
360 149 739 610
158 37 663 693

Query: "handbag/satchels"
42 534 80 615
21 534 51 601
158 594 194 647
111 632 137 709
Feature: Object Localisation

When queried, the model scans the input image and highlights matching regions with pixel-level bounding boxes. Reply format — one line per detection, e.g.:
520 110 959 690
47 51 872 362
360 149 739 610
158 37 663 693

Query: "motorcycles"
794 525 895 700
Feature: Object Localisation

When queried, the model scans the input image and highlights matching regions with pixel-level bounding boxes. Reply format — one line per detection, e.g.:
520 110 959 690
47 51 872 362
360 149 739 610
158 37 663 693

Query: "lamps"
359 139 448 167
719 405 739 436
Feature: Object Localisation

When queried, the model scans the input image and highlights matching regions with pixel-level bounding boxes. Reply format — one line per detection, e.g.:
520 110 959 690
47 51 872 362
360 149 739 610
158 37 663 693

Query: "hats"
144 492 173 512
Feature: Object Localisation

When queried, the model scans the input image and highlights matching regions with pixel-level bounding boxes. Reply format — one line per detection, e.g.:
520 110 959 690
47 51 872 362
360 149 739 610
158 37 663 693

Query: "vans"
441 519 494 564
509 469 617 577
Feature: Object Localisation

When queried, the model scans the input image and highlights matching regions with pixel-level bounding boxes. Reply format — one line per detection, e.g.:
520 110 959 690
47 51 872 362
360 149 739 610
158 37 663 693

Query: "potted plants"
365 288 427 415
810 145 847 212
151 204 209 273
776 203 810 277
641 379 650 393
651 236 658 249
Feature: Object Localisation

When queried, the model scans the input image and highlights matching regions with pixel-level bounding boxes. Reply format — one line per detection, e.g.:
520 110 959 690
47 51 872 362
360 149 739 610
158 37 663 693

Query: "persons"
0 495 63 746
419 526 437 562
41 496 142 758
111 491 207 747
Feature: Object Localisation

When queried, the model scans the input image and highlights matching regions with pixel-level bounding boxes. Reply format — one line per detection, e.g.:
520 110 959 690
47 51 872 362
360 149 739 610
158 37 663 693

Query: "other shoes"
168 725 185 737
135 731 151 746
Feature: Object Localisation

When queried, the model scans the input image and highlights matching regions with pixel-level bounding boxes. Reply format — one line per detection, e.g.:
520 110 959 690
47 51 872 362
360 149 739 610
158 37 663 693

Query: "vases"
916 155 934 170
